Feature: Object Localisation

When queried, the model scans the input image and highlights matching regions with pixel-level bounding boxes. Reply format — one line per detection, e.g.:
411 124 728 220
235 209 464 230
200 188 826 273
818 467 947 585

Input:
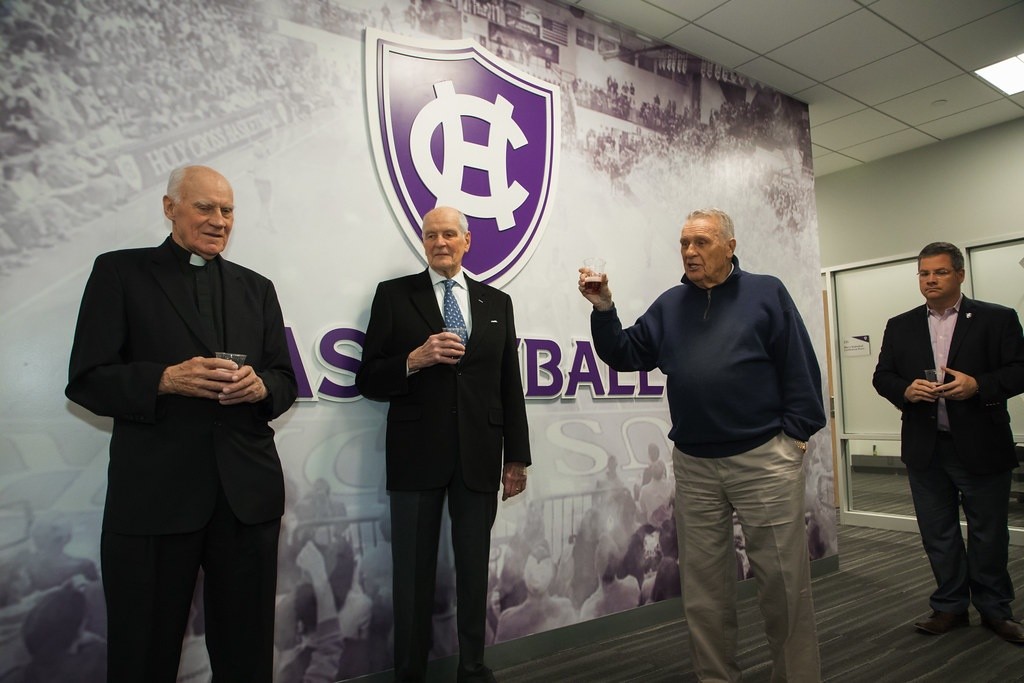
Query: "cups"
443 326 465 359
582 257 606 294
924 368 944 394
215 352 245 371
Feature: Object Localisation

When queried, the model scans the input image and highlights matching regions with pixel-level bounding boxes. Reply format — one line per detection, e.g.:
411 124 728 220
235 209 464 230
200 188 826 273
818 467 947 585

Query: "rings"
516 488 521 492
950 394 955 398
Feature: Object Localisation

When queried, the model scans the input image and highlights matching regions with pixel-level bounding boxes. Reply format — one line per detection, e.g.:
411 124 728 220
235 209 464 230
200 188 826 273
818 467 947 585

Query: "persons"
402 1 812 167
1 445 844 674
871 242 1024 646
354 207 534 682
65 164 300 683
571 209 825 683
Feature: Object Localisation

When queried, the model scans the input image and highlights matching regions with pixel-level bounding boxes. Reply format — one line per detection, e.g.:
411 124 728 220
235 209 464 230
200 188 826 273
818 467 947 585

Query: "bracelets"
793 440 807 453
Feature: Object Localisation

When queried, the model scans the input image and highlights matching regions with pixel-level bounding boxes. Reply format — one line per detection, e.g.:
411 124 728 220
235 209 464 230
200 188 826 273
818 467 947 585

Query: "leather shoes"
981 617 1024 643
913 614 969 635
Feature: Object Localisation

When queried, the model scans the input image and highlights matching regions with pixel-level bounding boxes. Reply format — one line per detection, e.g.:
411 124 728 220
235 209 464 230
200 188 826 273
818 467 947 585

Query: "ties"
442 279 468 361
